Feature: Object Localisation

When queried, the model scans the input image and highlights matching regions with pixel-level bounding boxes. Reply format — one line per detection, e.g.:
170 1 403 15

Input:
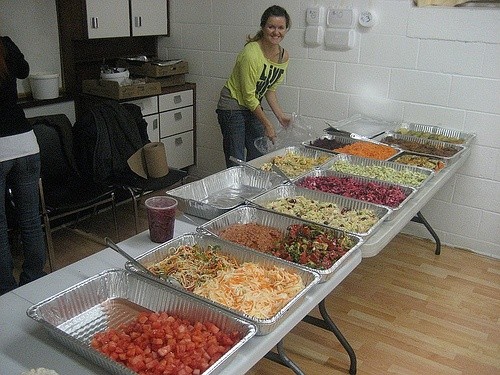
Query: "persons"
0 36 48 296
215 5 296 168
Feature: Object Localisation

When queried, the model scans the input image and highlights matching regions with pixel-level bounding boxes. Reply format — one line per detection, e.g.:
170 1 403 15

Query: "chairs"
24 104 191 272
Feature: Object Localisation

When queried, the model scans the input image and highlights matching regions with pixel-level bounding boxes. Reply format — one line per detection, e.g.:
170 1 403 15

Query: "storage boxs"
81 59 190 101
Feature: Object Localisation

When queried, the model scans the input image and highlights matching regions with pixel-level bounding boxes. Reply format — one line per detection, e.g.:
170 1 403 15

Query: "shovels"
105 238 183 288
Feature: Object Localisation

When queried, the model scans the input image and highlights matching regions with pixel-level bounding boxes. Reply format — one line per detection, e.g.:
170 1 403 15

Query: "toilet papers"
126 140 170 181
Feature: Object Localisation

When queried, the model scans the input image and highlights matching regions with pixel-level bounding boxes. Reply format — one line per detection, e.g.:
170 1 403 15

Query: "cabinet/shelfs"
73 82 198 170
82 0 171 40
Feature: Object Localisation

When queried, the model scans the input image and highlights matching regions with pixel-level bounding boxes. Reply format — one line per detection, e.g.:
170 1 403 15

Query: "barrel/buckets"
28 72 60 99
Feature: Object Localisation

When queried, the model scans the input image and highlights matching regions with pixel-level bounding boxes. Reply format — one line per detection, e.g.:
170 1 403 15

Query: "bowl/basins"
100 68 130 85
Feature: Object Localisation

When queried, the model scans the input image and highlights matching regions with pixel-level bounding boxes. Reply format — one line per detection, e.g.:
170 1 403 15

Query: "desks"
1 148 473 375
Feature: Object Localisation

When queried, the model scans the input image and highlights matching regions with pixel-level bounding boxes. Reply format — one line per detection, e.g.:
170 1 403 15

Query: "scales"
323 114 386 142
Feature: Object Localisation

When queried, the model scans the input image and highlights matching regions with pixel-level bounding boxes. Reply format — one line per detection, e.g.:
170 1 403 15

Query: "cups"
145 196 178 243
